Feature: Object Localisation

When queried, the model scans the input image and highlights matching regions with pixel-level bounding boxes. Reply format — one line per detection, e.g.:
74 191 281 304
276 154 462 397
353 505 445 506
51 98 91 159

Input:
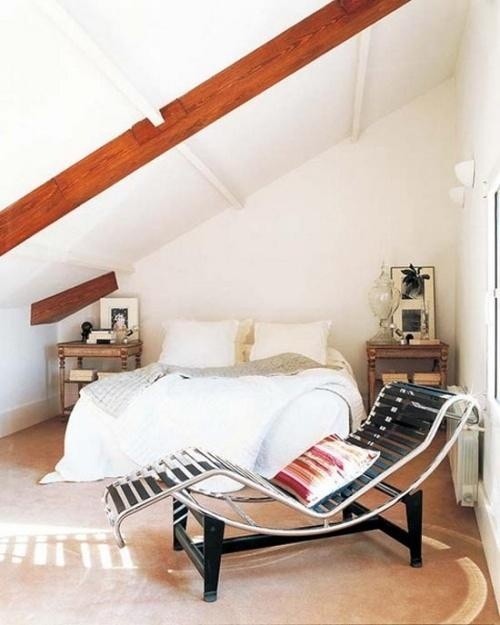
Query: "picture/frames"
99 297 140 343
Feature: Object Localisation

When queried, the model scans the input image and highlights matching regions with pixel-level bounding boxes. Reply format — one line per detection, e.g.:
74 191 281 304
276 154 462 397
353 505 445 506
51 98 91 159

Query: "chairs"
103 380 476 604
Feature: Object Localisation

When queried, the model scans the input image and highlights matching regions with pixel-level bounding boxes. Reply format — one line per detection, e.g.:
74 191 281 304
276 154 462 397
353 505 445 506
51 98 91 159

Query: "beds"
63 318 354 481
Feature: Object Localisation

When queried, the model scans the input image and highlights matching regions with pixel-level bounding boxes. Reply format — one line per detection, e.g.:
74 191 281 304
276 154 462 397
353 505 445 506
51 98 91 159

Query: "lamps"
449 158 476 210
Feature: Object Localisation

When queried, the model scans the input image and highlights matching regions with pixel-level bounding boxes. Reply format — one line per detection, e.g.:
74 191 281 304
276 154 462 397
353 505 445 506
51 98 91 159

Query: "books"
69 376 99 383
96 371 126 377
69 368 97 376
409 338 441 345
381 370 441 387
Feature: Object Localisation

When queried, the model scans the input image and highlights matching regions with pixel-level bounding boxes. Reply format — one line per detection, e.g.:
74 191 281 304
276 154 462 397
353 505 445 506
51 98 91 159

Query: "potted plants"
401 263 430 344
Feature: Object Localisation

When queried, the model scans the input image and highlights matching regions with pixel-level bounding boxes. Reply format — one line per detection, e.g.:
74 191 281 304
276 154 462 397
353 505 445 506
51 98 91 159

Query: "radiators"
446 384 482 510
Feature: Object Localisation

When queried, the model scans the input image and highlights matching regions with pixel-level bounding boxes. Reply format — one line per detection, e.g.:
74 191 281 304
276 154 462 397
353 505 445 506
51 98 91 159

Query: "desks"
365 339 450 414
56 339 143 422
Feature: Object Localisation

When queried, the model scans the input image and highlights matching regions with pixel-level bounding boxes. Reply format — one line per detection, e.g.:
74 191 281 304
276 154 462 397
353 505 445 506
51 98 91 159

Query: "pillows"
158 320 331 368
270 432 381 509
